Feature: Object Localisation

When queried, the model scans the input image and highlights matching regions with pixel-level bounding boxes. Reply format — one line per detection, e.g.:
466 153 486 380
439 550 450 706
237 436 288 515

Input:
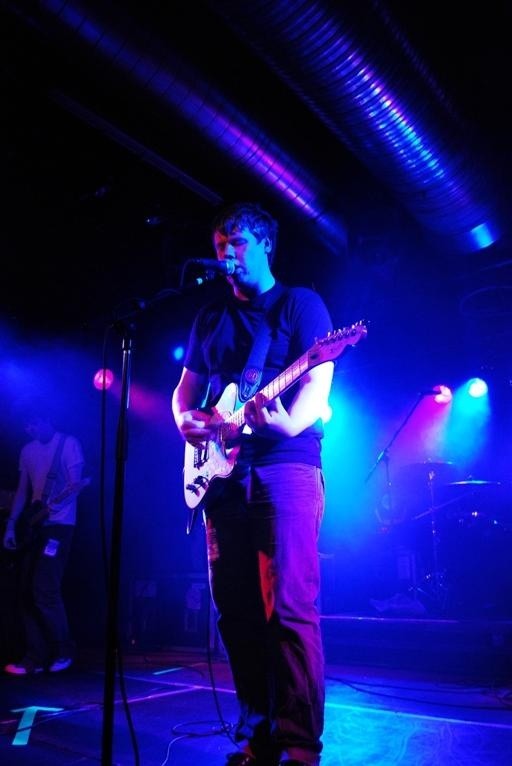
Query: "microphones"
194 253 239 279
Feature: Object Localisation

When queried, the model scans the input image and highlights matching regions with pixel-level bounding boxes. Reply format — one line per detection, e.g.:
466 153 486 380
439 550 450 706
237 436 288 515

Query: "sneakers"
5 649 77 678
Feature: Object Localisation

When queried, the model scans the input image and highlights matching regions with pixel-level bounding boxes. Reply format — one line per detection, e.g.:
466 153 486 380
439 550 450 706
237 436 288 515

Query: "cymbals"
401 458 457 468
443 479 498 487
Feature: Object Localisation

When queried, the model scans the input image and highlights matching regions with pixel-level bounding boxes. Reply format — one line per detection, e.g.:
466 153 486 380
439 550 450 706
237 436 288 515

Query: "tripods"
405 505 461 617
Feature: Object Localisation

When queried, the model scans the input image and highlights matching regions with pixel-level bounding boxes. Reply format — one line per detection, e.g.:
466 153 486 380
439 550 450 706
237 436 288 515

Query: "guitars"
182 320 368 510
2 471 90 565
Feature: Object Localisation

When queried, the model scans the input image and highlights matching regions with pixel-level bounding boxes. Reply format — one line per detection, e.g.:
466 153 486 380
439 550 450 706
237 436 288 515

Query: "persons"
168 206 335 765
1 408 85 673
